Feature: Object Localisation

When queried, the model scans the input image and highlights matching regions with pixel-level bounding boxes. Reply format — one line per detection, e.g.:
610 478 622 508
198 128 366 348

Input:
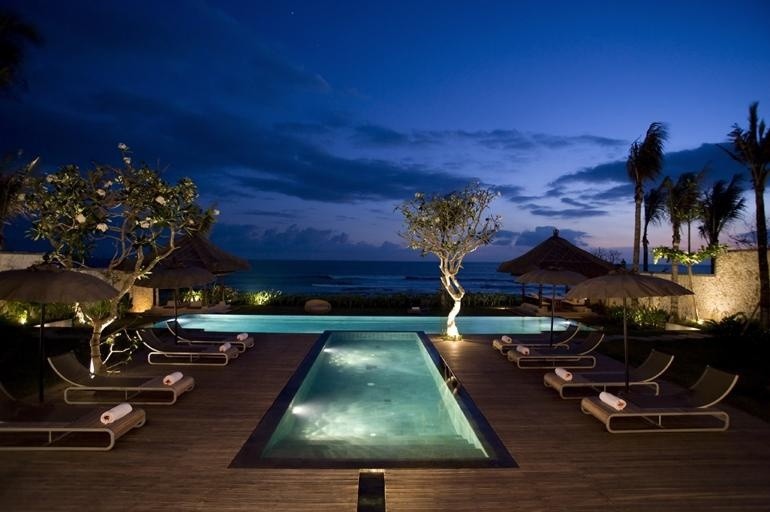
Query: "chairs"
494 324 579 356
543 349 675 399
49 351 195 405
138 328 239 367
166 320 254 353
507 332 606 370
0 386 146 453
581 365 739 433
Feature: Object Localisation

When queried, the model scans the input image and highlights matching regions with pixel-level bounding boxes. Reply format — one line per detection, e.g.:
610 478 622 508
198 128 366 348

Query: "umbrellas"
515 264 589 348
0 262 121 405
563 258 696 395
135 262 218 345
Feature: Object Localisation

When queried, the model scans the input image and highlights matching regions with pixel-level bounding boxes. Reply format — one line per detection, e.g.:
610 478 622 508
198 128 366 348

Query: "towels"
100 403 132 425
502 334 511 343
555 367 572 381
219 343 231 352
162 372 183 385
599 392 627 410
517 345 529 355
237 333 248 341
